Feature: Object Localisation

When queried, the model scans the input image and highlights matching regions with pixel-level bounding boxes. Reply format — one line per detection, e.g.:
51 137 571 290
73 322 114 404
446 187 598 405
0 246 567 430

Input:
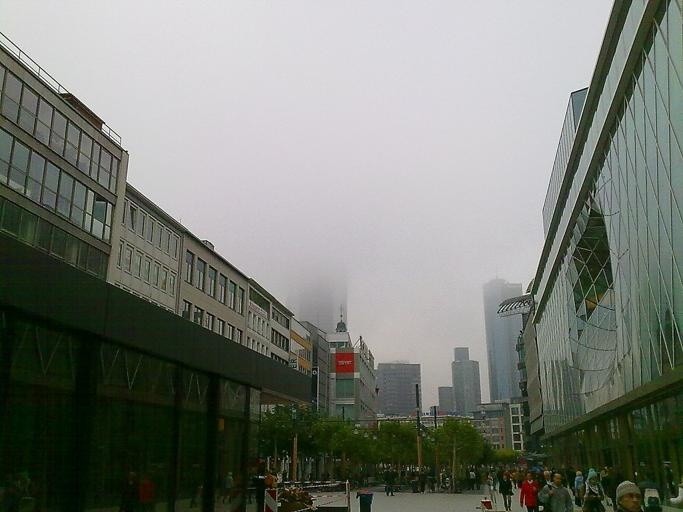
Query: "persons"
379 460 481 496
483 460 682 511
263 468 289 490
0 462 254 512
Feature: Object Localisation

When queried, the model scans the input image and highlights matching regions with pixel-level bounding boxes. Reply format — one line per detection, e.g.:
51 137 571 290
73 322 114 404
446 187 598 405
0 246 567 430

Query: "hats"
588 469 597 480
616 480 640 505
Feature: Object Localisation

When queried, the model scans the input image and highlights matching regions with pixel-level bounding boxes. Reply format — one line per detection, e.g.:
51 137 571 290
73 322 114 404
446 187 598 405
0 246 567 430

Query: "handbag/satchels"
540 485 552 512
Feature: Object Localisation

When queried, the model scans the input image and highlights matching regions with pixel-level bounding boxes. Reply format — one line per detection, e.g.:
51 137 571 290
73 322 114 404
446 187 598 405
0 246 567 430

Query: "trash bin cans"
356 493 374 512
